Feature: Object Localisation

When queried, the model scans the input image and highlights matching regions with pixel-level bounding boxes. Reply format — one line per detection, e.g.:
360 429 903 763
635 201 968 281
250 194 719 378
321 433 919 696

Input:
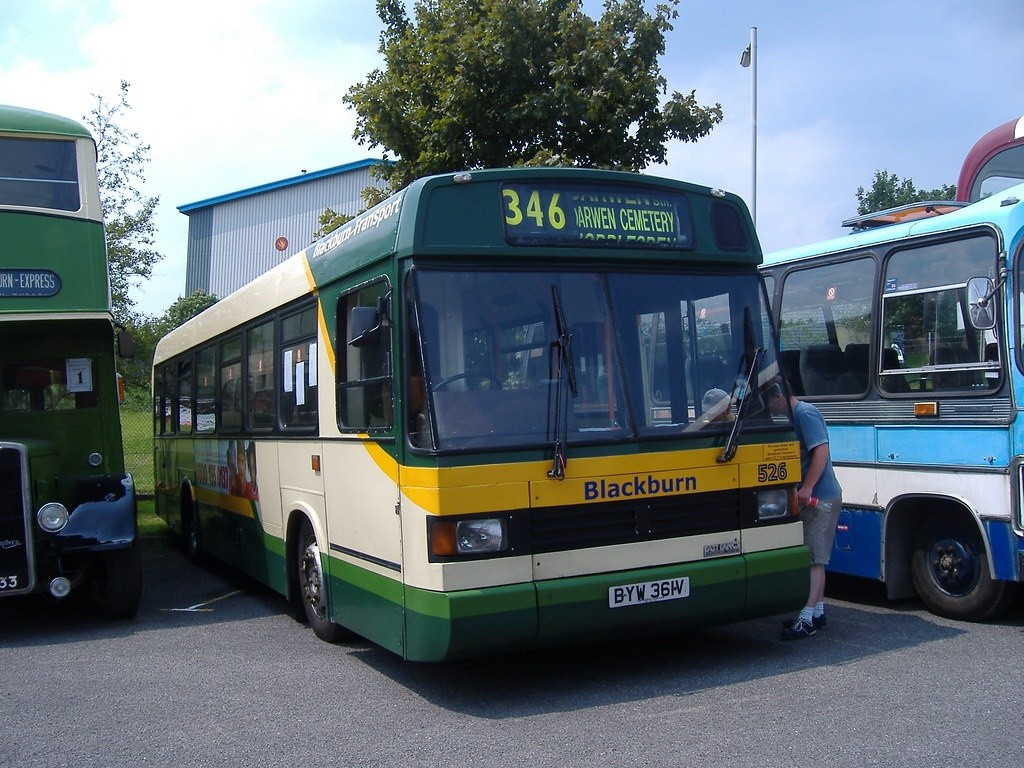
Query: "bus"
151 166 811 663
0 105 137 619
519 182 1024 621
955 115 1024 202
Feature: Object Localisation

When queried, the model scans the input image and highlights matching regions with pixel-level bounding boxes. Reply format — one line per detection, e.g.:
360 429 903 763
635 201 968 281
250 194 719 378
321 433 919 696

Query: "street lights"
739 27 757 229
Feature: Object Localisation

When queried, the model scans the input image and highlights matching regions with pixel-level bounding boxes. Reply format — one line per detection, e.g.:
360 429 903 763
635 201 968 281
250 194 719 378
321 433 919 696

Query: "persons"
758 376 842 640
702 388 737 422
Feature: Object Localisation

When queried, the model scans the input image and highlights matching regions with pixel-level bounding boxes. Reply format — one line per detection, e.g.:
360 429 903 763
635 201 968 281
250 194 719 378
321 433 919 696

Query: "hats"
701 387 732 413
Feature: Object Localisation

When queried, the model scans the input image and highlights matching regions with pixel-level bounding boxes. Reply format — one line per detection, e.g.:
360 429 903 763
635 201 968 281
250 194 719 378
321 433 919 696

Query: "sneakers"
778 615 817 639
781 608 829 628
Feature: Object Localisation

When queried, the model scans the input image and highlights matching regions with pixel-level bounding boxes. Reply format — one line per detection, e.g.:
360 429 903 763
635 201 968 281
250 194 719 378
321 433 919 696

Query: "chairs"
652 341 981 407
224 369 558 442
